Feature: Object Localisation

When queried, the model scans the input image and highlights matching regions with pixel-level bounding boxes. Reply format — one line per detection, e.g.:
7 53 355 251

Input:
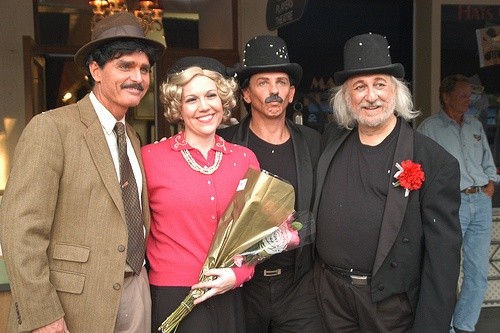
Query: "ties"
113 121 144 275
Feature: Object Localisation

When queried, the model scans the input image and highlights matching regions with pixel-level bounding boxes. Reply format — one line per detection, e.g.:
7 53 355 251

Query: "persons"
415 75 500 333
1 12 166 333
313 32 463 333
217 35 322 333
141 55 261 333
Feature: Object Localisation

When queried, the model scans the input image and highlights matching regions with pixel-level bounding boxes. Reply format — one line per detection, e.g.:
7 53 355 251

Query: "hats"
168 56 235 79
235 35 302 87
74 12 165 68
333 34 405 85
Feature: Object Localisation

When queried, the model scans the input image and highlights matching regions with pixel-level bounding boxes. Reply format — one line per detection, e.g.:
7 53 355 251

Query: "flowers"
233 213 302 267
392 160 425 196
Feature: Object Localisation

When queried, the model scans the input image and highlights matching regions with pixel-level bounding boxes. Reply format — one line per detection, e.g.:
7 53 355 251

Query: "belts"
462 185 486 193
323 264 372 287
